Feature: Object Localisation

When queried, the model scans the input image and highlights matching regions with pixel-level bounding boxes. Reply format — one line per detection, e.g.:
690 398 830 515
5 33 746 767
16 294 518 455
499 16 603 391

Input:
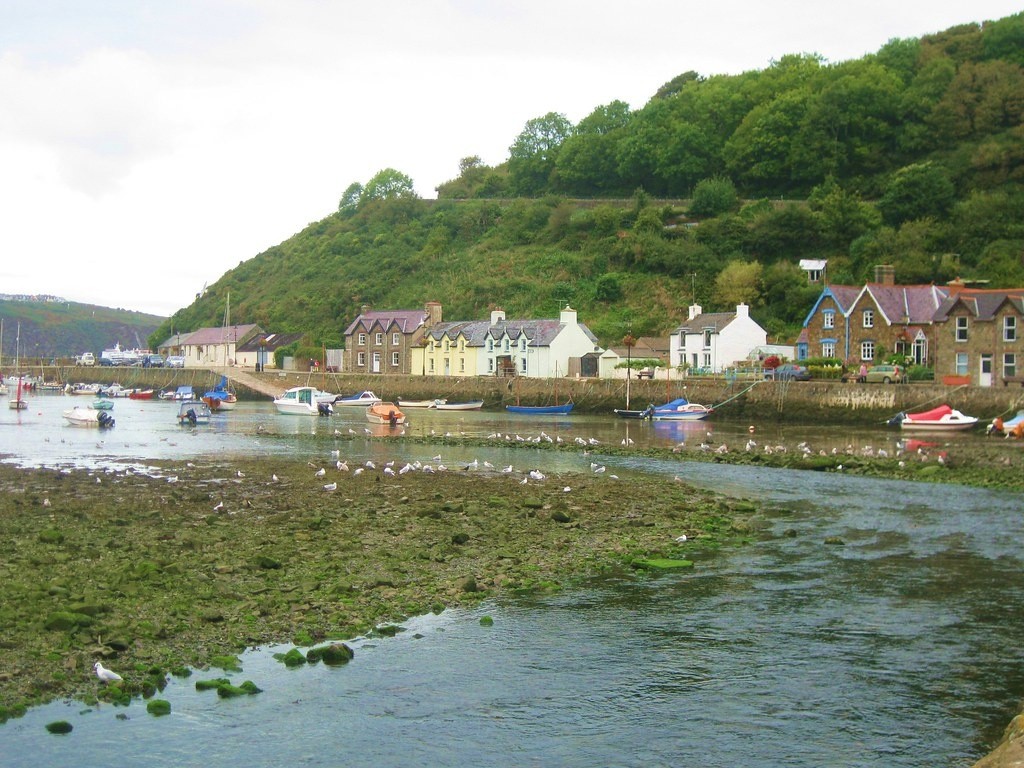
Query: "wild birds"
670 425 1012 485
36 403 638 513
94 662 123 688
675 534 687 544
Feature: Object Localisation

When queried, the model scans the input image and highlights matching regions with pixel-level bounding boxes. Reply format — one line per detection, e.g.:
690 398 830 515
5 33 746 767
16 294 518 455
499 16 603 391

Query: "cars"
99 359 117 367
862 364 908 384
167 356 184 367
765 362 813 382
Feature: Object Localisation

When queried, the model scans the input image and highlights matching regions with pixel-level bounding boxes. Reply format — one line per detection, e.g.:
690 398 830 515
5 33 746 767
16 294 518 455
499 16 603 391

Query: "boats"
884 404 980 431
433 396 486 411
301 390 343 404
987 408 1024 437
641 396 715 421
335 390 383 406
272 386 334 417
0 317 198 410
397 396 447 408
176 399 211 427
61 407 116 429
365 401 410 427
614 410 644 418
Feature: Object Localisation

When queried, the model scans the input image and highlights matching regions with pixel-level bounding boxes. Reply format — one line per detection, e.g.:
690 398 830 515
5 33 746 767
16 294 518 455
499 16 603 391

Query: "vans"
75 356 95 367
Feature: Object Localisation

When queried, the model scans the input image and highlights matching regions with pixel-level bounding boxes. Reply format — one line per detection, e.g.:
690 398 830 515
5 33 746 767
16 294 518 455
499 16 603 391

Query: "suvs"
146 355 165 368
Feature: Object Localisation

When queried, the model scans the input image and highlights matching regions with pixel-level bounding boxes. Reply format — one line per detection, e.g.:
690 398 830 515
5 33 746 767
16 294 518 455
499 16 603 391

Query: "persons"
861 362 867 383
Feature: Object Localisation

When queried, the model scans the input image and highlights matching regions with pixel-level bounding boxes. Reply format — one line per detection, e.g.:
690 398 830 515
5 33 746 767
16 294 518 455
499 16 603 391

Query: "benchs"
638 371 655 380
1003 374 1024 388
326 366 338 373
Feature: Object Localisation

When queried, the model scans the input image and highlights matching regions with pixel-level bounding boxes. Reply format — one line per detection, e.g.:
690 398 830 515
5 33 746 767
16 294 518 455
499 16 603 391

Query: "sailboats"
505 360 575 415
198 292 238 411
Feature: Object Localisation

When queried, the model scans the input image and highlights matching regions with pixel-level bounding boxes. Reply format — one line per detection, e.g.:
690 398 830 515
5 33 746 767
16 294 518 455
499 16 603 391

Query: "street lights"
175 328 181 356
421 324 427 376
259 333 265 373
626 318 634 379
